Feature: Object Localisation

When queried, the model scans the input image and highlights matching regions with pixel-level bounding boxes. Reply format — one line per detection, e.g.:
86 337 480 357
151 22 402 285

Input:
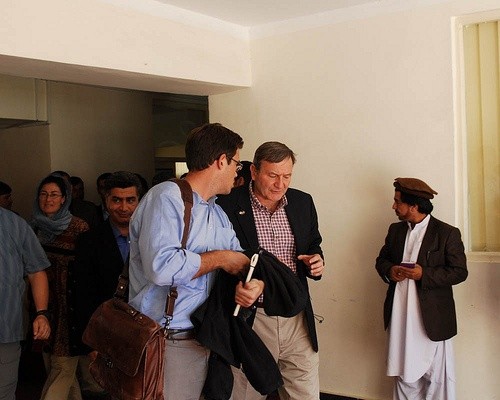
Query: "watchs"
35 309 50 319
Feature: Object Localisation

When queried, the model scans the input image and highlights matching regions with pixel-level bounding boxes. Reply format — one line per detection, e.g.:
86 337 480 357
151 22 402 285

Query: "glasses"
38 191 66 198
225 156 243 173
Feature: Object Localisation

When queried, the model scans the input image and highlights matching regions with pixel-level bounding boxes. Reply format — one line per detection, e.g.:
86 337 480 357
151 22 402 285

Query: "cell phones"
401 262 415 269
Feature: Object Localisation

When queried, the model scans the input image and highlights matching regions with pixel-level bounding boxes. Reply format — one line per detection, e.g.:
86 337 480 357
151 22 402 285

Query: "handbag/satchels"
83 298 167 400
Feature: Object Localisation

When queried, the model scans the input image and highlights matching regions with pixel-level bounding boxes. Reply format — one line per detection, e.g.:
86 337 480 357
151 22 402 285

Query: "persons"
49 170 70 193
68 176 99 228
375 178 468 399
26 176 90 400
0 182 20 216
125 122 264 400
0 207 52 400
97 173 113 200
69 170 144 361
214 141 326 400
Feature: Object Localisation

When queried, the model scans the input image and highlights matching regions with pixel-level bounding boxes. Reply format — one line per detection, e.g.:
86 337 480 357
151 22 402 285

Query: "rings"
398 272 401 276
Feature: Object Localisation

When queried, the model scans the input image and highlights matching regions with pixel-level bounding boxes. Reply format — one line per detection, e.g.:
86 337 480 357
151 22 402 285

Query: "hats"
393 176 438 199
